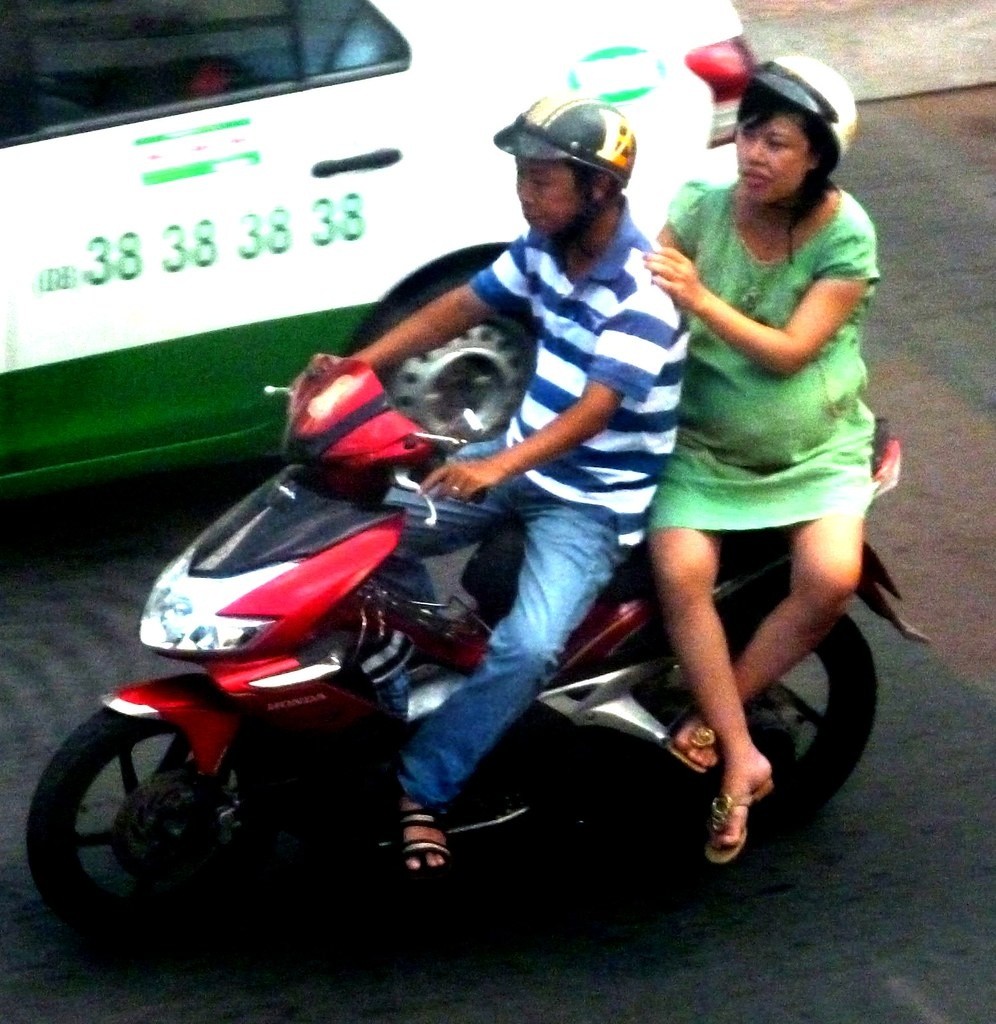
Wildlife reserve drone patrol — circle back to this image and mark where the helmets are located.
[495,93,636,189]
[754,50,858,160]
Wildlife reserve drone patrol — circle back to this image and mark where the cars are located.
[0,1,765,508]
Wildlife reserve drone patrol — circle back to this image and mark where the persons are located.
[314,96,689,881]
[643,54,877,862]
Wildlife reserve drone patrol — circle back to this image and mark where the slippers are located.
[705,776,775,864]
[665,715,722,773]
[385,793,452,880]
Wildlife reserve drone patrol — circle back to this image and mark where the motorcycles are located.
[25,349,933,934]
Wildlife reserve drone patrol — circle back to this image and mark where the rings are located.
[450,485,461,493]
